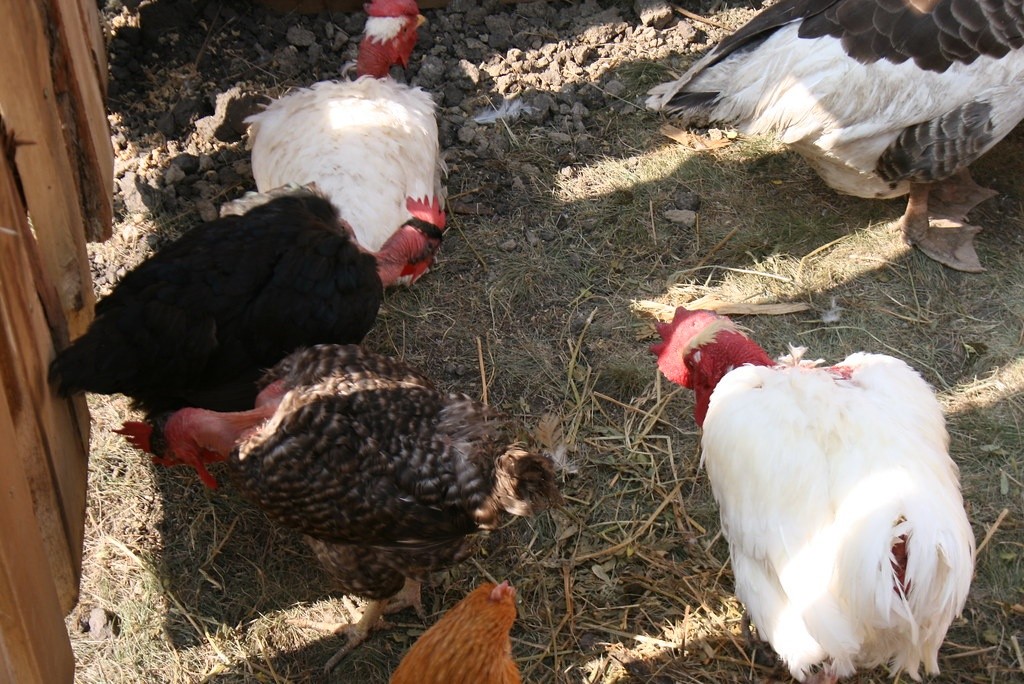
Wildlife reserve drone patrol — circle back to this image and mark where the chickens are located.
[387,579,523,684]
[648,305,978,684]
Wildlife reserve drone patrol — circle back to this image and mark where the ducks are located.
[644,0,1024,274]
